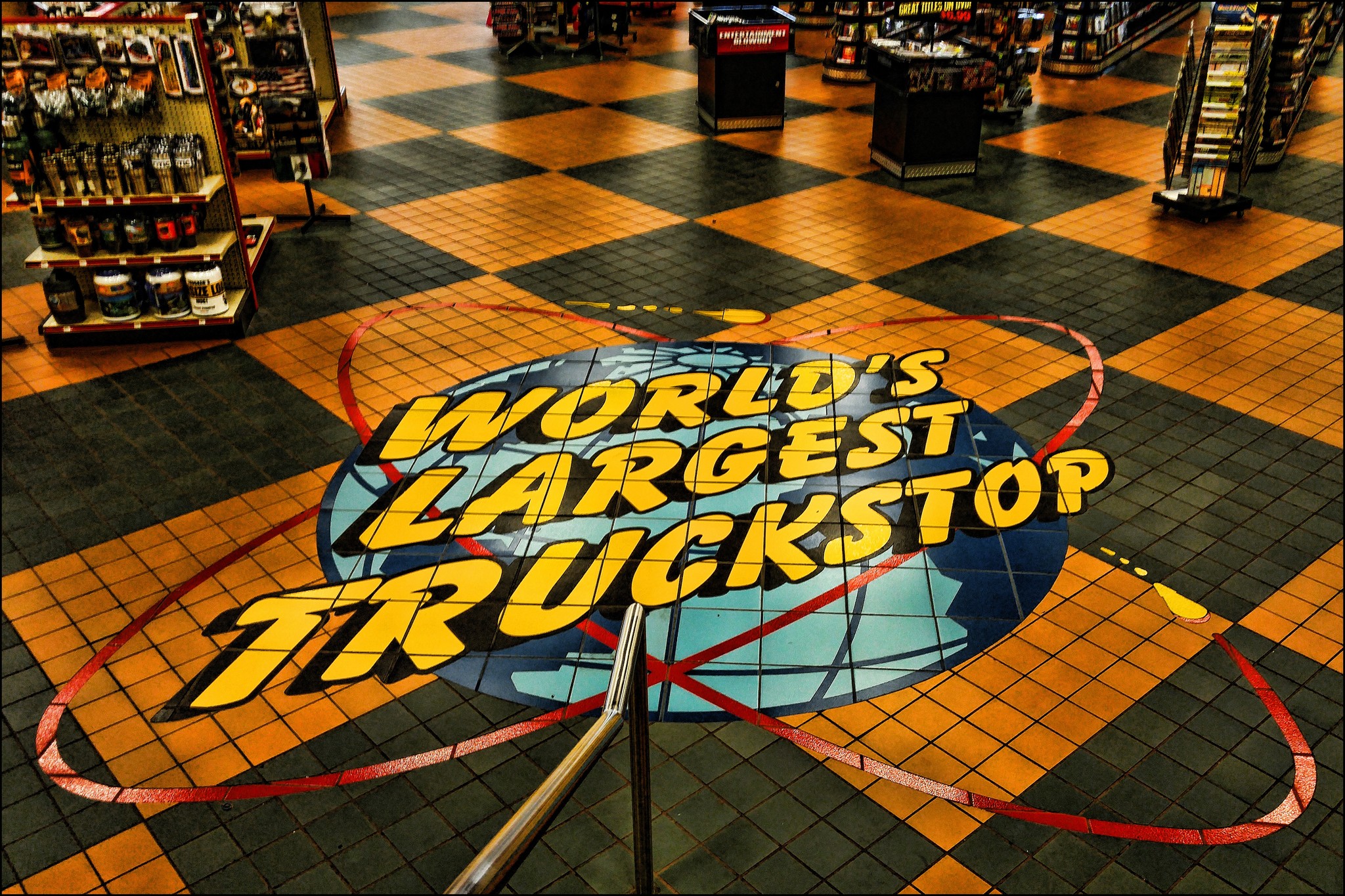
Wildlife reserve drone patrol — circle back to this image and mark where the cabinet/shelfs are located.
[821,2,961,84]
[1254,1,1344,173]
[1041,3,1201,79]
[0,17,276,345]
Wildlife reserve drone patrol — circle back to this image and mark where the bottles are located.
[97,207,125,254]
[153,204,206,253]
[30,207,65,251]
[93,265,142,321]
[145,264,192,318]
[184,260,230,317]
[43,267,87,325]
[58,208,97,257]
[125,205,153,254]
[41,134,206,197]
